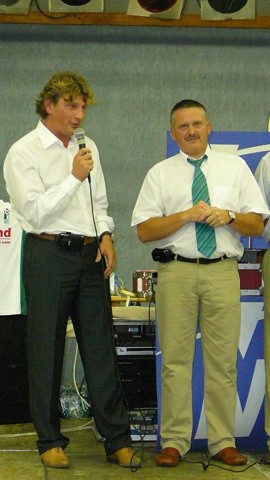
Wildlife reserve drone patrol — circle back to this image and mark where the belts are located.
[33,232,95,245]
[172,253,227,265]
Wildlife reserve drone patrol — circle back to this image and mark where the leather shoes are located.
[155,448,180,466]
[41,446,69,468]
[213,447,248,465]
[107,446,141,467]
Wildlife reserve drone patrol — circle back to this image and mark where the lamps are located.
[197,0,257,20]
[126,0,191,20]
[48,0,105,13]
[0,0,35,15]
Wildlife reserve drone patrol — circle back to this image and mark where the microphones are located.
[74,127,91,182]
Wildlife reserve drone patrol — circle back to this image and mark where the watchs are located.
[99,231,115,244]
[227,210,235,224]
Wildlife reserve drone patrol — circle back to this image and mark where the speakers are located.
[0,314,34,424]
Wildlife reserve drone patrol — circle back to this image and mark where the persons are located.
[1,72,141,468]
[253,153,270,464]
[131,99,270,466]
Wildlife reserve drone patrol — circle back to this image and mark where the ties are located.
[187,154,217,258]
